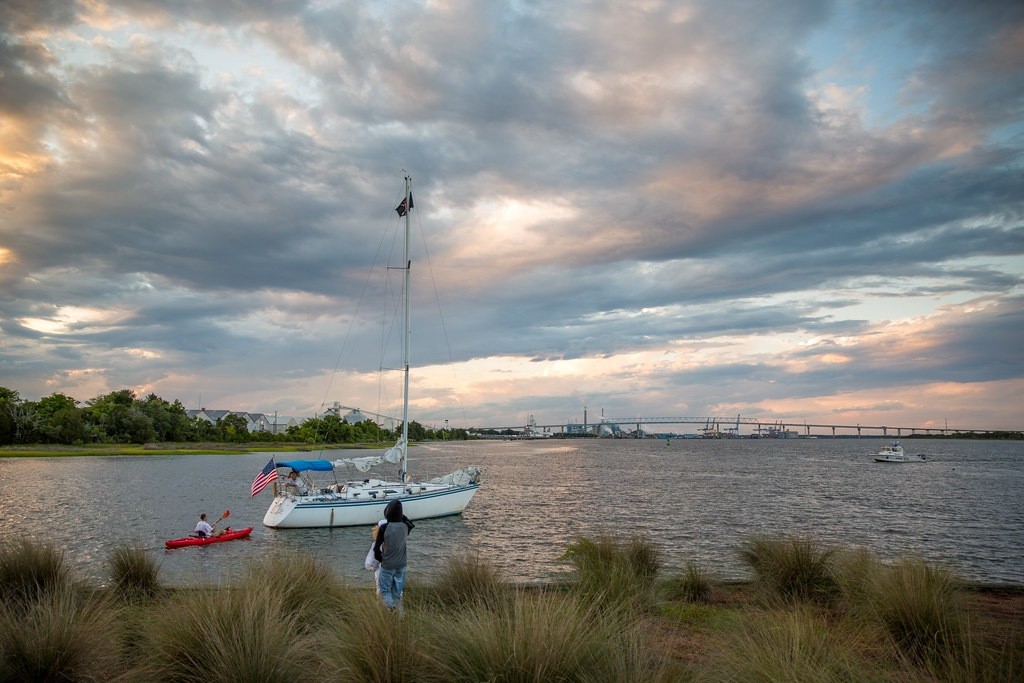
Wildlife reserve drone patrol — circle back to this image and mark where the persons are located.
[194,514,227,538]
[364,499,415,622]
[284,468,308,496]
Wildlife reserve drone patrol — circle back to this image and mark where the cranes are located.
[696,413,742,435]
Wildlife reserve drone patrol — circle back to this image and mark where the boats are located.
[868,441,930,463]
[165,526,255,548]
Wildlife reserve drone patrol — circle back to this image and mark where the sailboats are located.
[262,170,487,529]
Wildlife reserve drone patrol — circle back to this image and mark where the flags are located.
[248,457,279,498]
[395,190,414,217]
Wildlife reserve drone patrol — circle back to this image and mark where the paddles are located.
[211,510,230,526]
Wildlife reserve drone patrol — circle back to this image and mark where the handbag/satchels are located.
[365,541,382,571]
